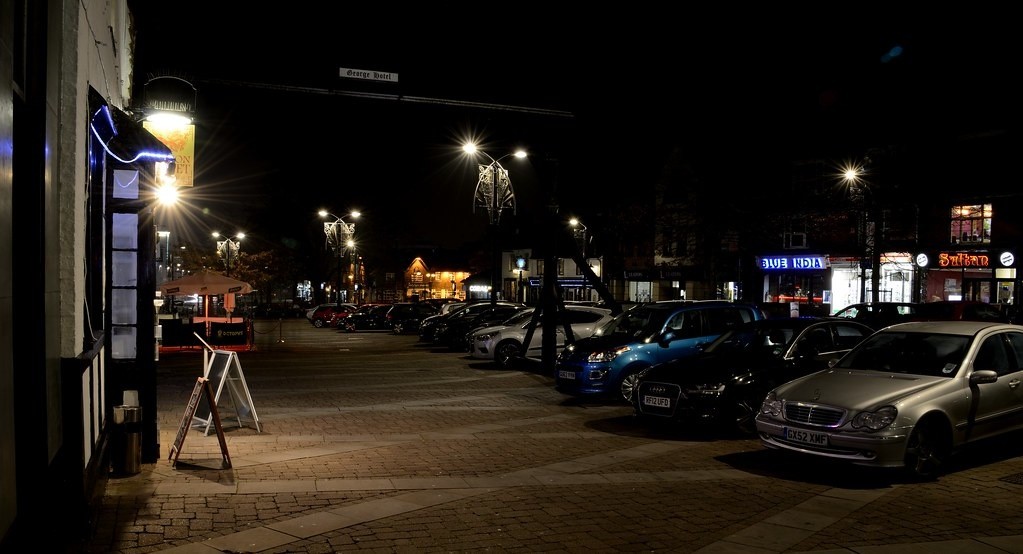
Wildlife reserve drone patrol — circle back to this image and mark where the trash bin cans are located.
[112,406,142,475]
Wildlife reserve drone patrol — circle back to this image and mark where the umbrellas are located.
[160,268,253,338]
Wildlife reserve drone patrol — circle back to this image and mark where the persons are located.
[978,230,989,238]
[267,292,275,311]
[931,290,941,302]
[306,292,308,299]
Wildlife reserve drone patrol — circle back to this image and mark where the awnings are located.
[90,84,175,166]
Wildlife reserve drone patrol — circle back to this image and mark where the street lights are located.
[317,207,363,302]
[570,217,589,266]
[213,231,244,277]
[463,139,532,300]
[842,164,883,304]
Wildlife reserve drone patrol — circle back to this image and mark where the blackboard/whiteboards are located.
[172,377,232,457]
[194,351,258,423]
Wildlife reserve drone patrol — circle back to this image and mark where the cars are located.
[837,300,1011,330]
[553,297,766,403]
[469,305,616,369]
[633,315,883,430]
[306,296,528,346]
[756,322,1023,476]
